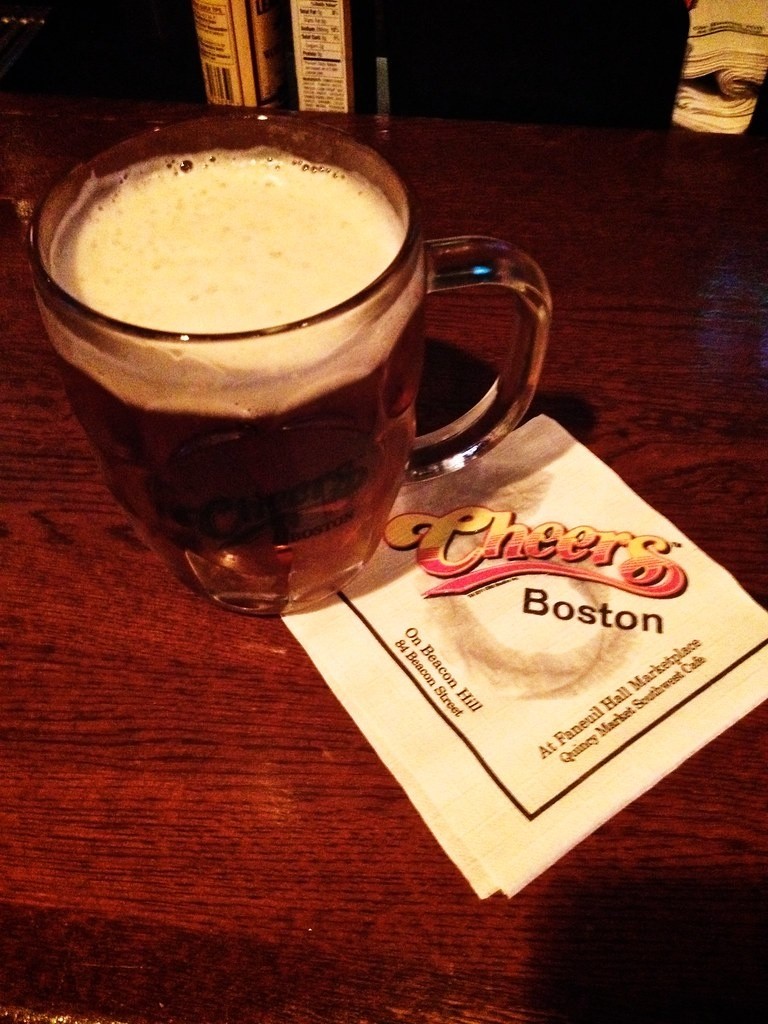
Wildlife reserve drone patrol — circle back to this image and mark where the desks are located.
[0,91,767,1024]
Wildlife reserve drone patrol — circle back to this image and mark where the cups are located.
[26,107,552,615]
